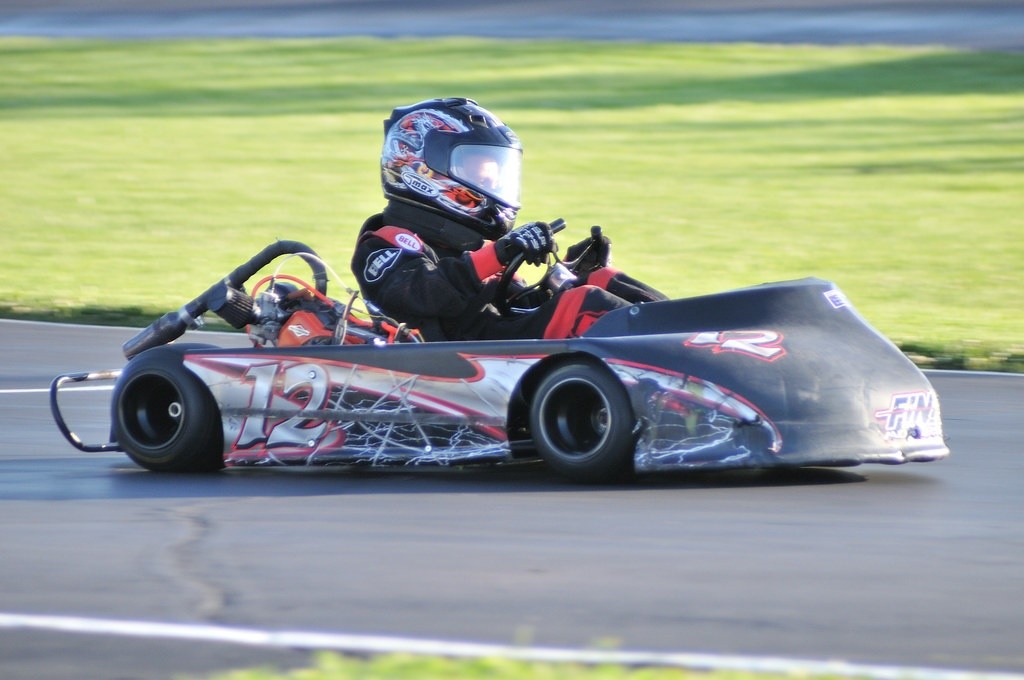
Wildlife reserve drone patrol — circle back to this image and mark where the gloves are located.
[567,234,612,273]
[494,222,559,266]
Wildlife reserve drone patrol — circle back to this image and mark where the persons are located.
[352,96,671,341]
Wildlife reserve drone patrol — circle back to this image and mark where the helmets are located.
[379,96,523,240]
